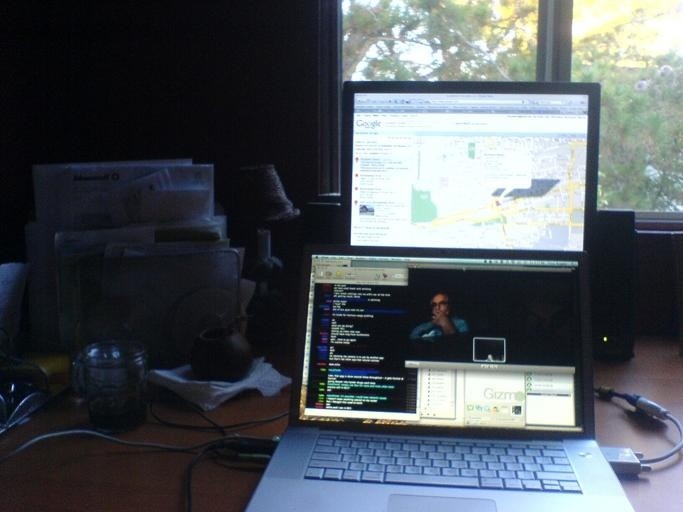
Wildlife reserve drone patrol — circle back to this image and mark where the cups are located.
[72,339,149,434]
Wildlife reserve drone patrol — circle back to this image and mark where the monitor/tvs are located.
[339,80,601,256]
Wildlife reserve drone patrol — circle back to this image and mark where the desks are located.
[0,332,683,512]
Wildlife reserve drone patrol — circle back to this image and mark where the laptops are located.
[242,245,636,512]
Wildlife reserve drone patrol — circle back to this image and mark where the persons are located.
[410,287,475,365]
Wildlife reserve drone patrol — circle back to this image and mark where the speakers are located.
[593,209,636,361]
[303,200,341,246]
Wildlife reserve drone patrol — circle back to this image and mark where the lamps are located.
[233,162,301,283]
